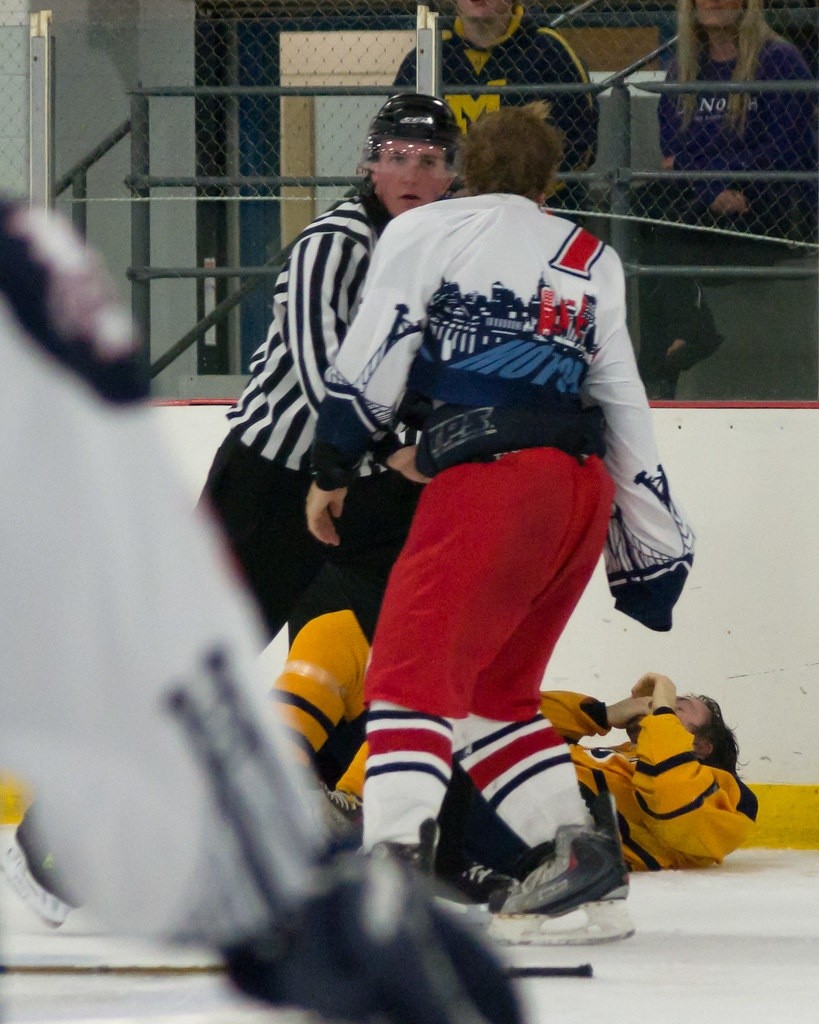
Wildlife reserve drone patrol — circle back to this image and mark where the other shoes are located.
[636,324,726,379]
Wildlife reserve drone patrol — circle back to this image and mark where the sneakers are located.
[434,842,519,915]
[5,799,85,927]
[489,823,634,949]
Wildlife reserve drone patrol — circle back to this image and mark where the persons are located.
[192,92,467,721]
[302,112,690,942]
[267,606,760,914]
[623,1,815,405]
[382,1,598,221]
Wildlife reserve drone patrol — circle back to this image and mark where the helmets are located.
[361,90,462,180]
